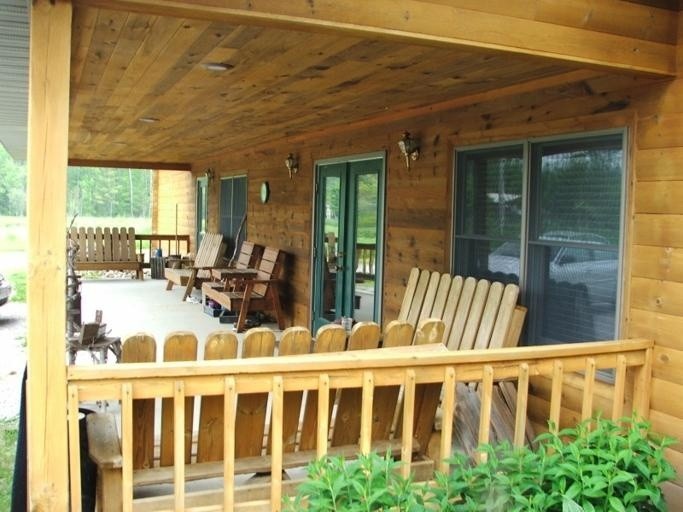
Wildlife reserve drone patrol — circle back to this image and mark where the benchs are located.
[346,266,529,433]
[86,320,450,512]
[66,226,143,280]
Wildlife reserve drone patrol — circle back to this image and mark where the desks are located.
[68,336,122,409]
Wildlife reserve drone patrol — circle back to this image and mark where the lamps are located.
[285,152,298,179]
[397,129,420,169]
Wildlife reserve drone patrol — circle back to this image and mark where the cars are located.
[488,229,618,314]
[0,272,12,305]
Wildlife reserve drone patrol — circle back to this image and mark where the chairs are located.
[201,240,286,334]
[162,232,224,302]
